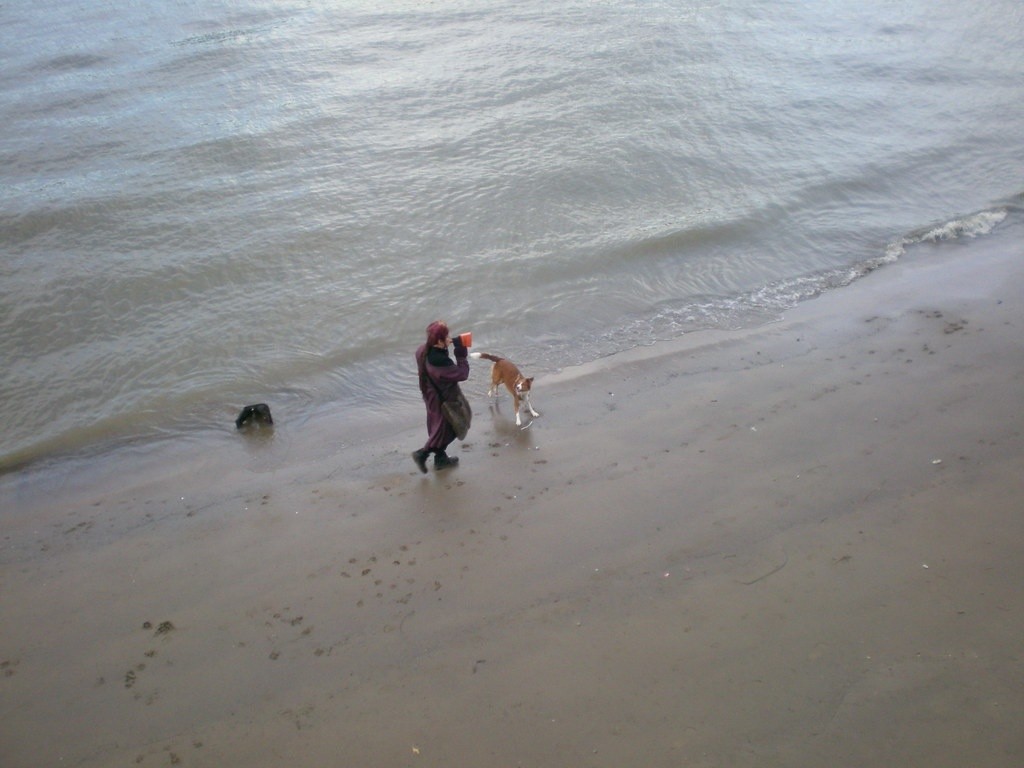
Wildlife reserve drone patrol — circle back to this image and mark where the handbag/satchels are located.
[439,385,473,442]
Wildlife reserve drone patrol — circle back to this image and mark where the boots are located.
[411,450,430,474]
[433,451,459,471]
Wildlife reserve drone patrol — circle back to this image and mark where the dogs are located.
[471,353,539,426]
[236,403,273,425]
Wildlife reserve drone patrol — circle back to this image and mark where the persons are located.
[410,320,469,475]
